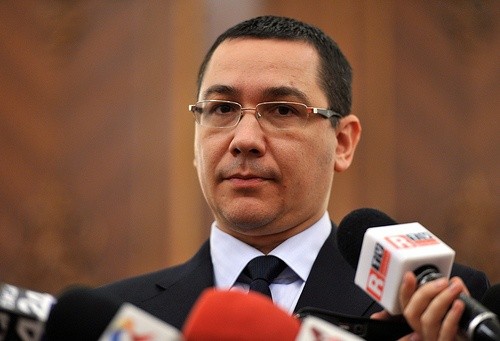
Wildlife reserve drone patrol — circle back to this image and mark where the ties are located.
[242,255,287,303]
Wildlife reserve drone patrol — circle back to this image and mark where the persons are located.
[90,15,491,341]
[398,271,487,341]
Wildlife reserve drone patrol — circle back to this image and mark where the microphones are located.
[0,208,500,341]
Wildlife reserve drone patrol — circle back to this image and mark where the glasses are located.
[189,100,342,129]
